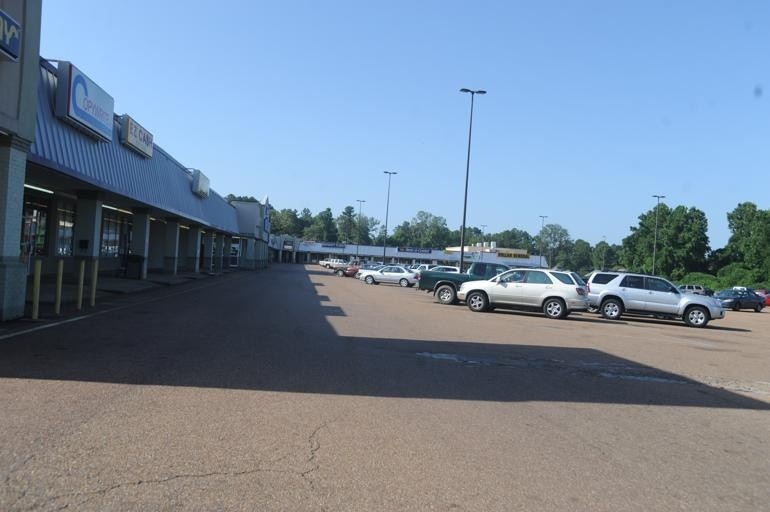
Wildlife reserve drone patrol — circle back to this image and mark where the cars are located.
[457,268,590,319]
[589,271,770,328]
[319,258,460,287]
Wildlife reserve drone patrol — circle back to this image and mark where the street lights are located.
[539,215,547,268]
[357,200,366,256]
[384,171,398,264]
[459,88,487,273]
[652,195,665,275]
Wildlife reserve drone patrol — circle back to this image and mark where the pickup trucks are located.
[418,261,512,304]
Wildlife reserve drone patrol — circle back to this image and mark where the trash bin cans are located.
[126,255,144,279]
